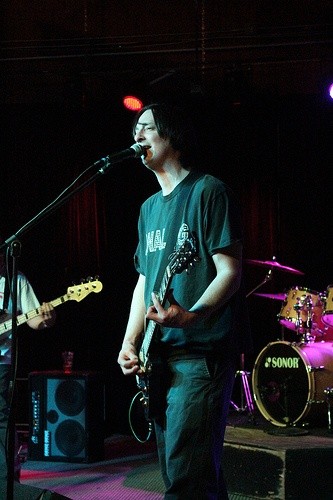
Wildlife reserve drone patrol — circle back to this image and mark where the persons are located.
[0,230,53,482]
[117,104,246,500]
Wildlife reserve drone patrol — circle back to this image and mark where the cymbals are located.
[254,292,287,301]
[246,259,304,275]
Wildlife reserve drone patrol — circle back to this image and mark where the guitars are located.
[131,239,198,409]
[0,279,104,346]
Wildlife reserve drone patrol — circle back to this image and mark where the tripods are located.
[228,355,254,426]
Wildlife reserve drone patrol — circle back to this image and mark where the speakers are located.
[39,374,105,463]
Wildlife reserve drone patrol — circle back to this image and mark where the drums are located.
[252,341,333,428]
[277,286,325,335]
[314,310,333,343]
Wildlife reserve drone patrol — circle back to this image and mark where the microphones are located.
[95,143,144,165]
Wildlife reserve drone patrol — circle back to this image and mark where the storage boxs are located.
[223,426,333,500]
